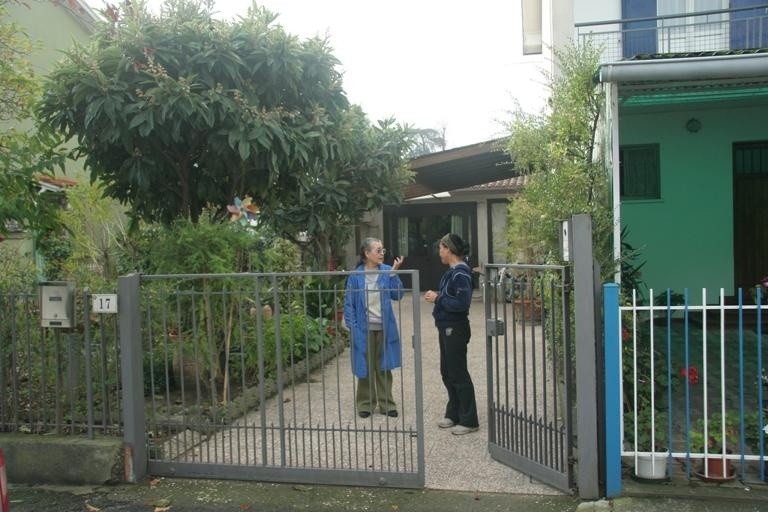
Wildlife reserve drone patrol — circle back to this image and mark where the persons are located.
[425,233,480,435]
[342,236,404,417]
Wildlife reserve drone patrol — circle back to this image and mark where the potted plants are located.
[504,193,547,324]
[623,406,672,484]
[326,217,352,275]
[687,409,762,483]
[146,205,252,388]
[651,289,685,320]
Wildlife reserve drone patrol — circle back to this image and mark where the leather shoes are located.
[382,408,398,418]
[358,408,370,418]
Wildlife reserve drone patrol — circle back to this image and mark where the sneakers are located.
[450,424,479,435]
[436,417,458,428]
